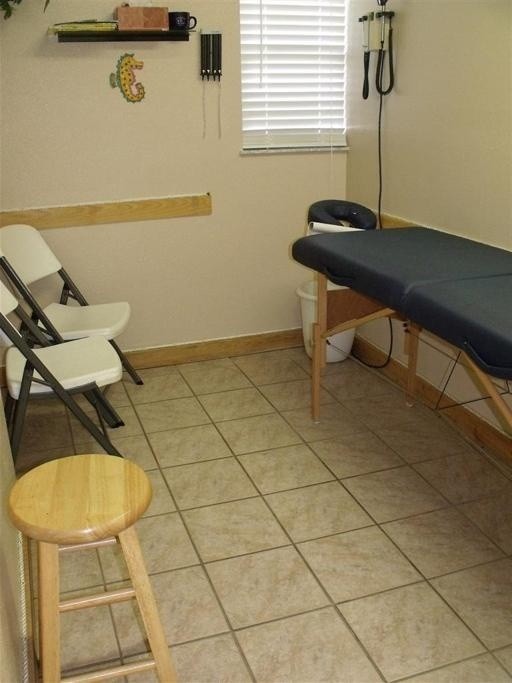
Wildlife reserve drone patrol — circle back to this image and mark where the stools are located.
[9,454,178,683]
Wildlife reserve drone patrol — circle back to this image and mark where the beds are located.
[292,227,512,435]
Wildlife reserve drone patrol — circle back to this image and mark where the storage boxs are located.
[115,7,169,30]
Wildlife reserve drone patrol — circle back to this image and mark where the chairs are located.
[0,223,144,427]
[0,279,123,463]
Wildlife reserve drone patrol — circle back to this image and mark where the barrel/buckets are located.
[295,280,356,363]
